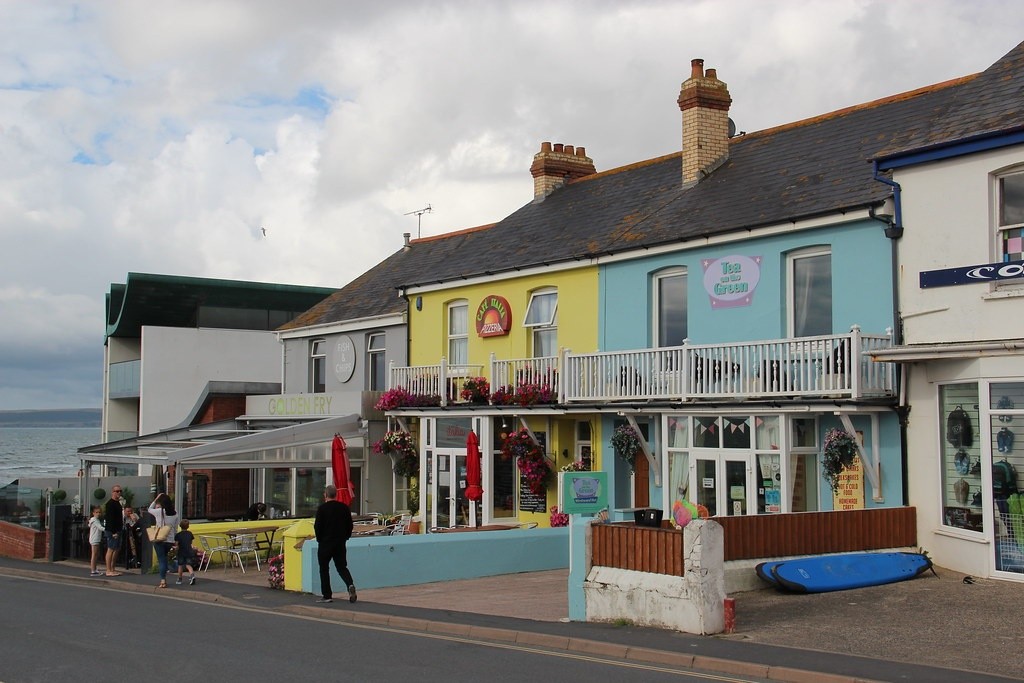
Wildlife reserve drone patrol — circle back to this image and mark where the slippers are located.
[954,451,969,474]
[954,480,969,503]
[997,430,1014,452]
[996,399,1014,421]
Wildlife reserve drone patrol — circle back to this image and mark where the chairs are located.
[431,524,513,532]
[361,513,413,536]
[427,524,471,532]
[273,525,292,554]
[516,521,539,529]
[199,534,261,575]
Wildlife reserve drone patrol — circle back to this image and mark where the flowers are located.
[373,384,442,410]
[560,461,590,473]
[371,430,415,456]
[819,426,859,495]
[608,424,640,475]
[550,505,569,527]
[459,365,559,409]
[502,426,557,497]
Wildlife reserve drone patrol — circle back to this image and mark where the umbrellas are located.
[464,429,485,526]
[331,431,356,505]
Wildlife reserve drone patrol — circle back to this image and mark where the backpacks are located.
[947,406,973,446]
[993,458,1017,498]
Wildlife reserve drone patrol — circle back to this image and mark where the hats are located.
[971,491,982,505]
[970,462,980,473]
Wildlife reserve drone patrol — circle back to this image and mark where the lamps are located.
[502,416,508,428]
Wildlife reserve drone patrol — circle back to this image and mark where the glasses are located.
[113,490,122,493]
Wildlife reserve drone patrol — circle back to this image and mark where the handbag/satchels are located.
[146,508,170,543]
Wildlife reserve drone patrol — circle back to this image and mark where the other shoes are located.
[189,576,195,584]
[91,571,103,577]
[106,570,123,576]
[176,578,182,584]
[160,580,167,587]
[348,585,357,603]
[316,595,333,602]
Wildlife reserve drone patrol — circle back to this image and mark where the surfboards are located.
[771,552,934,593]
[755,559,807,592]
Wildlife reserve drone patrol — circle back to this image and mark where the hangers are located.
[955,405,963,412]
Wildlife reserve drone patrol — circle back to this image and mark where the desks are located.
[696,356,740,394]
[224,526,279,567]
[353,524,387,536]
[352,515,374,523]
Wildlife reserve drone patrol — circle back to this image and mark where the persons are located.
[121,504,141,565]
[104,485,123,576]
[174,519,195,585]
[314,485,358,603]
[247,502,267,520]
[88,507,106,576]
[148,493,179,588]
